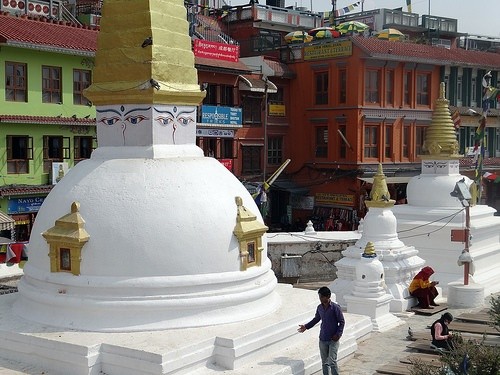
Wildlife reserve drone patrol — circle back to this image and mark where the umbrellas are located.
[285,21,404,41]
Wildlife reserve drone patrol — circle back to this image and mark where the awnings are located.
[0,210,14,230]
[360,176,416,184]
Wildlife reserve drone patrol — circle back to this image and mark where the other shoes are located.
[431,301,439,306]
[421,304,434,309]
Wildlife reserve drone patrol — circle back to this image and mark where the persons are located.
[281,211,305,232]
[298,286,345,375]
[431,312,455,351]
[409,266,440,309]
